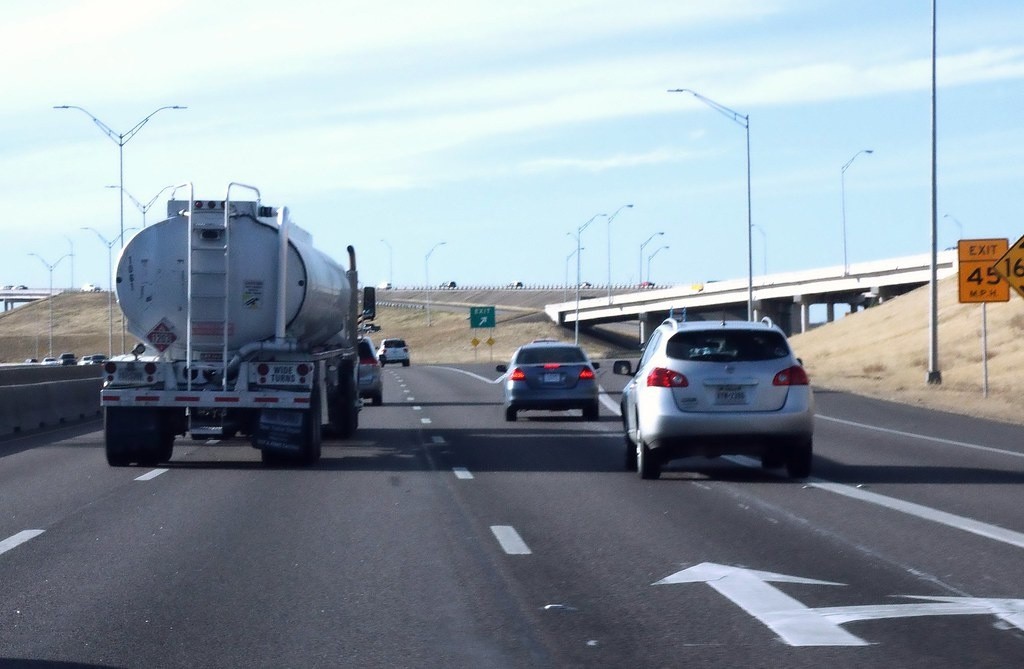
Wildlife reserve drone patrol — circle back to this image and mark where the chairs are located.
[668,342,691,360]
[735,340,766,358]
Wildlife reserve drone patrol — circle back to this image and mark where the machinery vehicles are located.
[99,180,375,463]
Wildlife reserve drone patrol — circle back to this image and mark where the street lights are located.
[80,225,140,357]
[53,101,187,356]
[574,213,606,345]
[607,204,634,305]
[565,249,585,302]
[941,214,964,244]
[647,245,670,288]
[667,87,752,323]
[378,238,394,290]
[104,183,176,228]
[425,240,447,330]
[28,252,74,358]
[841,148,875,272]
[640,231,664,288]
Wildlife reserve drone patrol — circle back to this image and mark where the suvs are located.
[375,339,410,367]
[439,281,456,290]
[353,337,385,405]
[612,317,815,482]
[377,282,391,291]
[496,343,601,421]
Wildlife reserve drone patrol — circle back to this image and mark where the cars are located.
[81,283,101,292]
[638,281,653,288]
[580,282,591,288]
[508,281,522,287]
[12,284,28,290]
[22,353,108,367]
